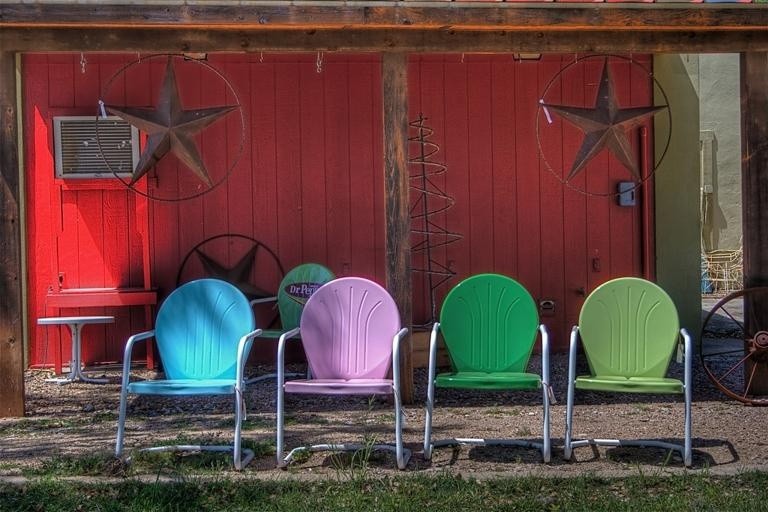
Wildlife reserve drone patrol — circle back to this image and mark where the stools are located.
[38,314,115,384]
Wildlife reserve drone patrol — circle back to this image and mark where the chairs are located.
[242,262,339,386]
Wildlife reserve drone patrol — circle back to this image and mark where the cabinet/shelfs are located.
[47,285,158,376]
